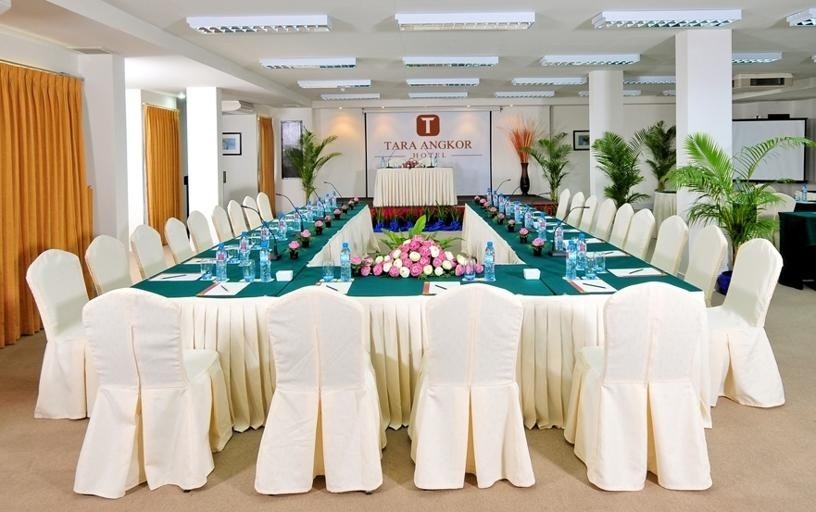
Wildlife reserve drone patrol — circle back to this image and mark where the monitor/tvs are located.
[731,118,808,184]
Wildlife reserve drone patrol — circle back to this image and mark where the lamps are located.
[408,92,468,99]
[320,93,381,101]
[783,8,816,28]
[539,54,641,67]
[406,78,480,88]
[259,57,357,71]
[401,56,499,70]
[511,77,587,86]
[296,79,372,89]
[186,14,334,35]
[495,90,555,99]
[579,90,641,97]
[623,74,676,87]
[394,11,536,32]
[591,8,742,30]
[733,51,782,64]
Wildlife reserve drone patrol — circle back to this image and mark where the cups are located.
[585,257,597,280]
[596,253,606,272]
[201,262,214,281]
[243,260,257,282]
[323,260,335,281]
[464,258,476,280]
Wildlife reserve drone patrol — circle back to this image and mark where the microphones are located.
[324,181,343,206]
[423,147,435,168]
[276,193,304,232]
[524,192,552,214]
[386,146,398,168]
[240,205,282,261]
[505,186,520,204]
[309,187,326,217]
[496,179,511,192]
[549,207,590,256]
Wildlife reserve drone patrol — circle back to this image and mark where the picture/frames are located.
[222,132,242,156]
[573,130,590,151]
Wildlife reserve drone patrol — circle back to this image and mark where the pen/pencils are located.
[327,284,337,292]
[435,283,446,290]
[629,269,644,274]
[602,251,614,255]
[197,258,213,262]
[584,283,606,289]
[220,284,228,292]
[162,273,187,278]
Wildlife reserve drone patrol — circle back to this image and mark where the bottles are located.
[524,206,533,229]
[577,233,588,275]
[801,184,808,200]
[216,242,226,280]
[325,194,330,213]
[331,191,338,209]
[554,221,564,252]
[538,213,547,243]
[307,201,313,222]
[515,201,520,222]
[259,242,271,282]
[506,197,511,215]
[317,197,323,217]
[381,157,385,168]
[566,241,577,280]
[486,188,491,203]
[341,242,352,282]
[434,153,438,167]
[294,208,301,231]
[493,191,498,210]
[278,213,287,240]
[485,241,495,282]
[240,231,250,266]
[260,221,269,247]
[499,193,505,212]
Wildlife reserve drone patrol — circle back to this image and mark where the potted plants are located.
[421,203,435,230]
[495,116,547,195]
[405,206,417,230]
[389,205,402,232]
[448,202,462,230]
[374,201,383,231]
[663,132,816,295]
[434,199,449,229]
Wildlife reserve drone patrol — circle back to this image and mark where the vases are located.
[498,219,503,225]
[343,209,347,213]
[290,251,298,260]
[520,236,527,244]
[325,222,331,228]
[316,229,322,235]
[302,240,309,248]
[533,246,542,256]
[508,225,514,232]
[335,215,340,220]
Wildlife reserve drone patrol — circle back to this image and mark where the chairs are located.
[623,208,655,261]
[683,225,727,307]
[211,206,233,242]
[243,196,262,230]
[578,194,598,233]
[765,192,795,219]
[256,192,274,222]
[564,282,712,492]
[74,288,214,498]
[26,248,90,420]
[608,203,634,250]
[85,234,132,295]
[255,284,382,494]
[131,225,167,278]
[708,238,784,408]
[164,217,193,265]
[187,210,213,253]
[649,215,689,277]
[566,191,585,228]
[407,284,536,489]
[555,188,571,222]
[227,200,248,237]
[590,198,617,242]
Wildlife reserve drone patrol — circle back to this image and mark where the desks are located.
[465,200,704,428]
[777,210,815,289]
[131,202,368,432]
[277,264,555,429]
[794,199,815,211]
[375,166,452,206]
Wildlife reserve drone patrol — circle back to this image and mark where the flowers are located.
[333,209,342,215]
[288,241,300,252]
[348,200,354,204]
[519,228,529,236]
[497,213,505,219]
[489,207,497,212]
[325,215,331,222]
[473,195,480,200]
[351,215,484,279]
[315,220,323,229]
[507,219,515,226]
[354,196,359,201]
[484,202,490,207]
[341,205,348,209]
[480,199,486,204]
[401,158,418,169]
[532,238,545,248]
[300,229,311,240]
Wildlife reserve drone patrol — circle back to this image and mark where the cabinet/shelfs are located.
[501,193,556,216]
